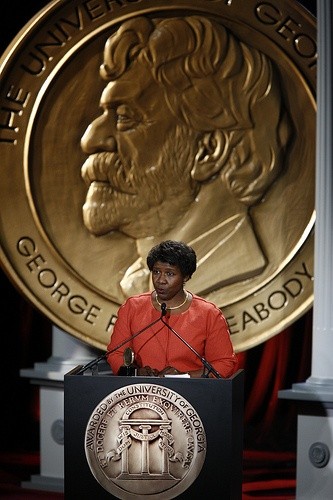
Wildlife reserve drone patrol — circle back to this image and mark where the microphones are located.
[76,303,224,378]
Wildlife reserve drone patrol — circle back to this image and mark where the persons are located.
[107,240,238,379]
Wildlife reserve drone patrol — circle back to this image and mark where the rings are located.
[174,370,178,373]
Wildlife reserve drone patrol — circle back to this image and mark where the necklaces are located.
[156,289,188,310]
